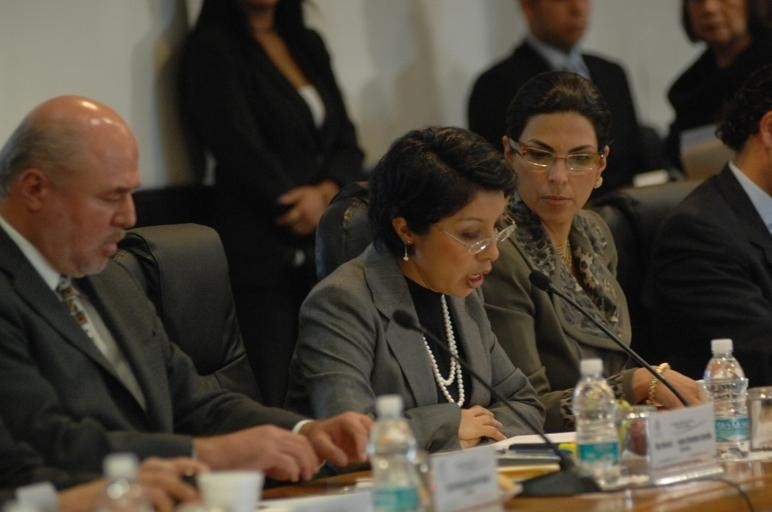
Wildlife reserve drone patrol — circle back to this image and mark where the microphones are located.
[528,266,691,409]
[390,306,596,497]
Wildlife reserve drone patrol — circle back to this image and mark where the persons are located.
[0,456,209,512]
[282,127,546,452]
[0,95,379,491]
[469,2,647,197]
[169,2,366,416]
[483,71,702,432]
[640,70,771,386]
[659,1,772,176]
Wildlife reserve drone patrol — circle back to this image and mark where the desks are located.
[178,391,770,508]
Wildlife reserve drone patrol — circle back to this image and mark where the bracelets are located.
[648,354,668,405]
[112,465,152,512]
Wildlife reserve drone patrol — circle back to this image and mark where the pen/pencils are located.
[508,442,577,450]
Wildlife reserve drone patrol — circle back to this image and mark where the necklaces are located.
[418,291,462,384]
[429,293,467,411]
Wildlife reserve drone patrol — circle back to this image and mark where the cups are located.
[621,404,657,486]
[749,385,772,454]
[194,470,265,512]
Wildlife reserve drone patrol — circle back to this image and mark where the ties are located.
[56,281,112,358]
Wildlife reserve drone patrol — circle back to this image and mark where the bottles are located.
[15,481,61,512]
[703,338,746,484]
[100,452,154,511]
[368,394,420,510]
[573,360,621,488]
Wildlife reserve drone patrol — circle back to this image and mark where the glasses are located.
[509,132,605,171]
[434,212,518,257]
